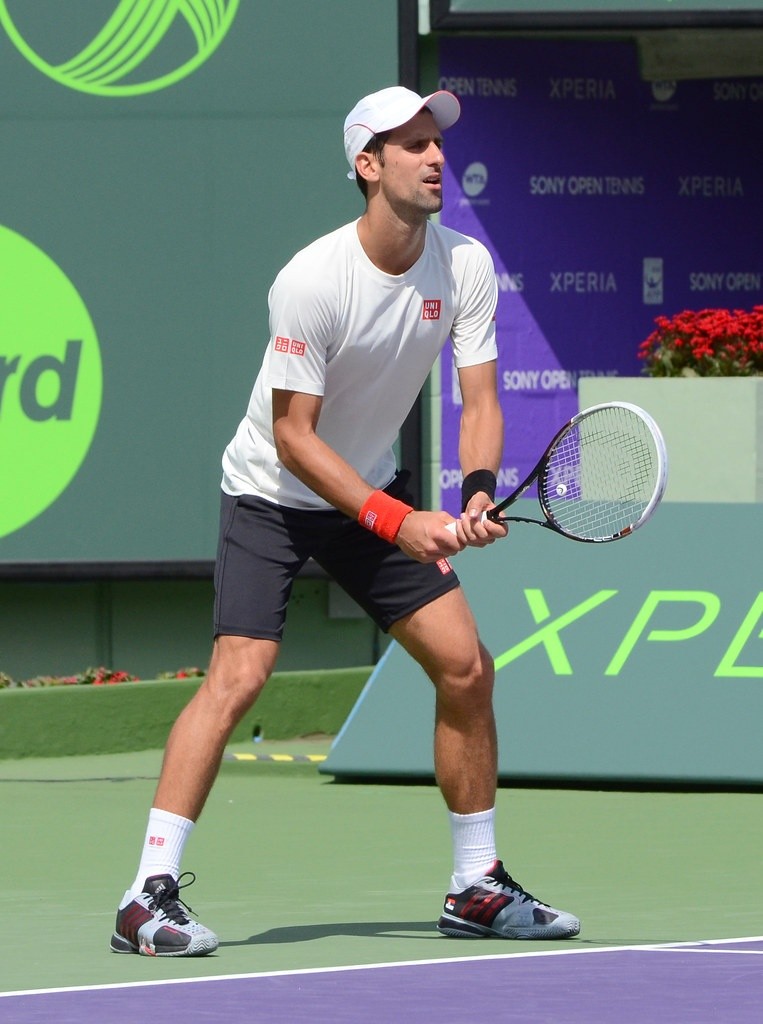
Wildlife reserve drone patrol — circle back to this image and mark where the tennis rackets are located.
[445,402,669,544]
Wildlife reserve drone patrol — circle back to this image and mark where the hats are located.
[342,86,461,182]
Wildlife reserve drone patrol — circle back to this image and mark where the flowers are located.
[639,306,763,378]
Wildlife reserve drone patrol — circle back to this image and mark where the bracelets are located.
[359,490,414,544]
[460,470,497,514]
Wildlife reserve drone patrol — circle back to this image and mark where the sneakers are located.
[437,859,581,941]
[109,875,220,956]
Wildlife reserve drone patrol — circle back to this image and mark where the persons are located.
[110,85,582,958]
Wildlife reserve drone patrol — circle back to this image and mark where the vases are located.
[576,376,763,503]
